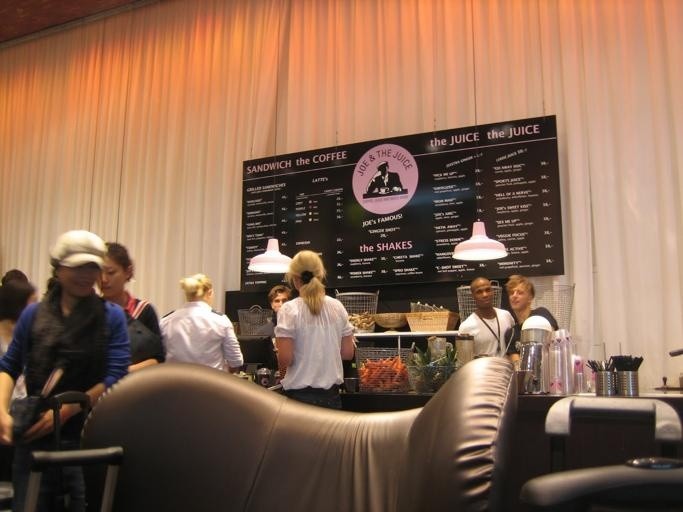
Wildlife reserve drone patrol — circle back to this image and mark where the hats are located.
[50,230,107,269]
[376,161,388,169]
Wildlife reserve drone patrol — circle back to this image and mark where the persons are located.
[505,275,561,367]
[268,286,293,318]
[457,276,516,366]
[0,229,130,511]
[159,274,244,376]
[274,250,355,409]
[364,162,405,196]
[1,279,37,405]
[96,243,165,375]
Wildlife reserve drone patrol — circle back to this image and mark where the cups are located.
[546,330,573,394]
[575,372,586,393]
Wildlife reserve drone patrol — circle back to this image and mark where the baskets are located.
[405,311,460,333]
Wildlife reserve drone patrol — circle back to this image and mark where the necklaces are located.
[476,309,502,351]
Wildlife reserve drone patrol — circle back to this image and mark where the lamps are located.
[452,222,508,261]
[248,239,293,274]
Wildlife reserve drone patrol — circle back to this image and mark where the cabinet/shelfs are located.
[227,334,488,413]
